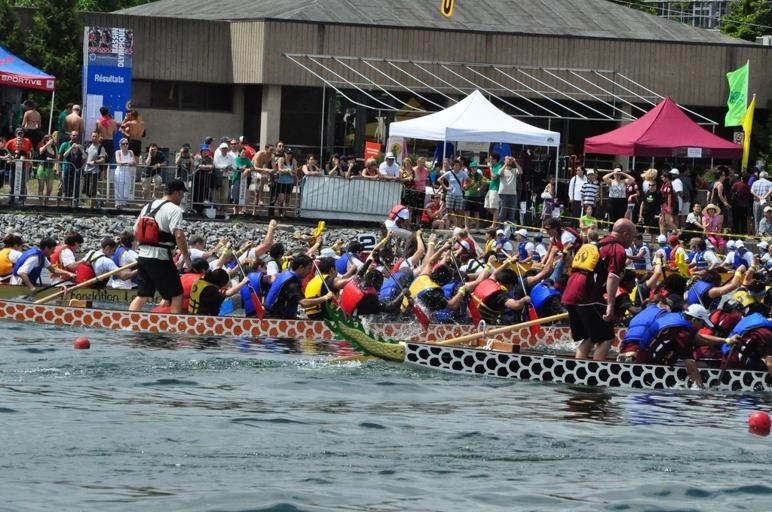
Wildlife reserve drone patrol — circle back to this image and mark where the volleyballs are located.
[74,336,90,349]
[750,412,771,430]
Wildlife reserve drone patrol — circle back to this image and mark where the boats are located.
[0,297,633,359]
[310,290,772,400]
[0,277,165,306]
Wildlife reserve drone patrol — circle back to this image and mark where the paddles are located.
[380,258,430,332]
[514,261,544,335]
[449,251,480,328]
[230,246,266,321]
[15,280,71,301]
[322,306,569,367]
[313,261,341,309]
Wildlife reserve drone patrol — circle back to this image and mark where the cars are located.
[561,165,615,181]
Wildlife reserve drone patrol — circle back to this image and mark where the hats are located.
[685,304,717,328]
[448,219,769,271]
[167,180,189,192]
[201,136,440,179]
[431,194,440,199]
[469,163,477,168]
[569,161,771,221]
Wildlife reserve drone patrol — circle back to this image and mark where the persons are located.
[747,410,771,436]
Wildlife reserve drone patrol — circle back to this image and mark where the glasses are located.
[120,143,129,146]
